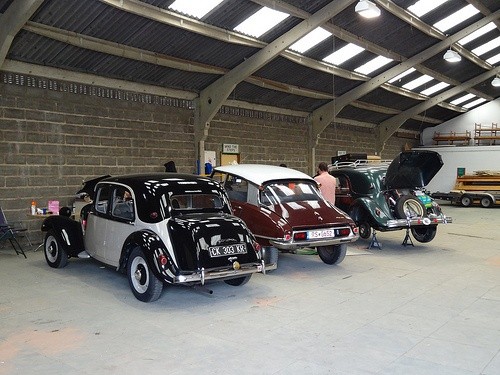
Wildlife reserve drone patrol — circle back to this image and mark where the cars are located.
[312,149,453,243]
[40,172,266,303]
[207,163,361,265]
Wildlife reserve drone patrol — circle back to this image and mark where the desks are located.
[28,212,72,252]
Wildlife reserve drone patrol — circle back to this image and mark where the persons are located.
[313,162,339,206]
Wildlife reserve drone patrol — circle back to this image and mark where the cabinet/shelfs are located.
[473,123,500,146]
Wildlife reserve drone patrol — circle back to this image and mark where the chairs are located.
[0,206,29,258]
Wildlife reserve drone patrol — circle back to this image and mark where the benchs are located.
[431,130,471,146]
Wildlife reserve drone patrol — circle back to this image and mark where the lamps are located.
[492,74,499,87]
[444,49,462,63]
[354,0,382,18]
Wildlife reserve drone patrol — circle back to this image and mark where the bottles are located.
[31,202,35,215]
